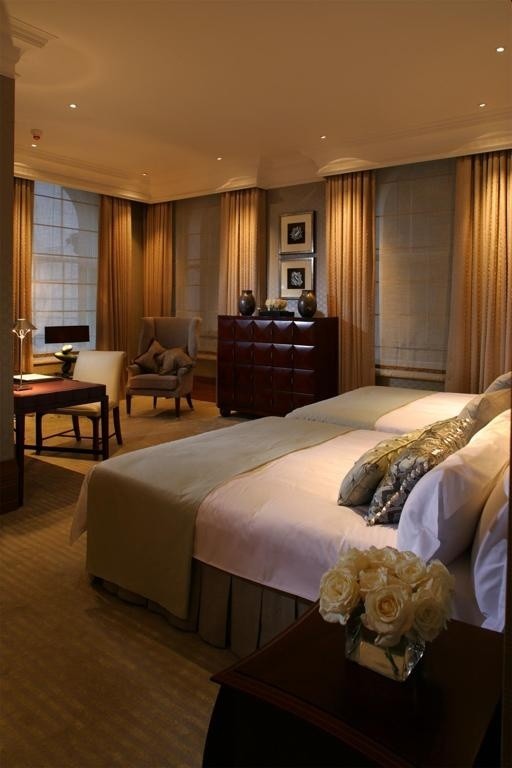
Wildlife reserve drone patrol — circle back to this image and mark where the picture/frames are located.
[277,254,317,301]
[278,209,317,256]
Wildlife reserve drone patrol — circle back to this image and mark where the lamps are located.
[10,316,38,391]
[43,324,90,380]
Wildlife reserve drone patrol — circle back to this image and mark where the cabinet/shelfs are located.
[215,313,340,420]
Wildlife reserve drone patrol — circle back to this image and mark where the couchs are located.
[125,312,202,418]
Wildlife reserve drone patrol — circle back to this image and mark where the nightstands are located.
[199,594,512,767]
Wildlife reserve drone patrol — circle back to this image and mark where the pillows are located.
[336,432,422,509]
[471,464,510,634]
[395,409,511,577]
[149,347,195,374]
[460,389,511,424]
[132,336,164,371]
[368,412,479,524]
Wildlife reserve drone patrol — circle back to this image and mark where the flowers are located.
[318,542,460,643]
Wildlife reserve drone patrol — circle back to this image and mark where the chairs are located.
[35,348,127,456]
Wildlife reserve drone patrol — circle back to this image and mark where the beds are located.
[284,383,512,435]
[64,412,512,660]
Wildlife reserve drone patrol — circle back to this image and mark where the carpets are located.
[0,393,252,765]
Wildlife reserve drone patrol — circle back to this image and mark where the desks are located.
[10,373,112,507]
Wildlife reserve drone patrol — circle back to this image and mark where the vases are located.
[338,619,429,683]
[237,289,256,316]
[297,289,318,318]
